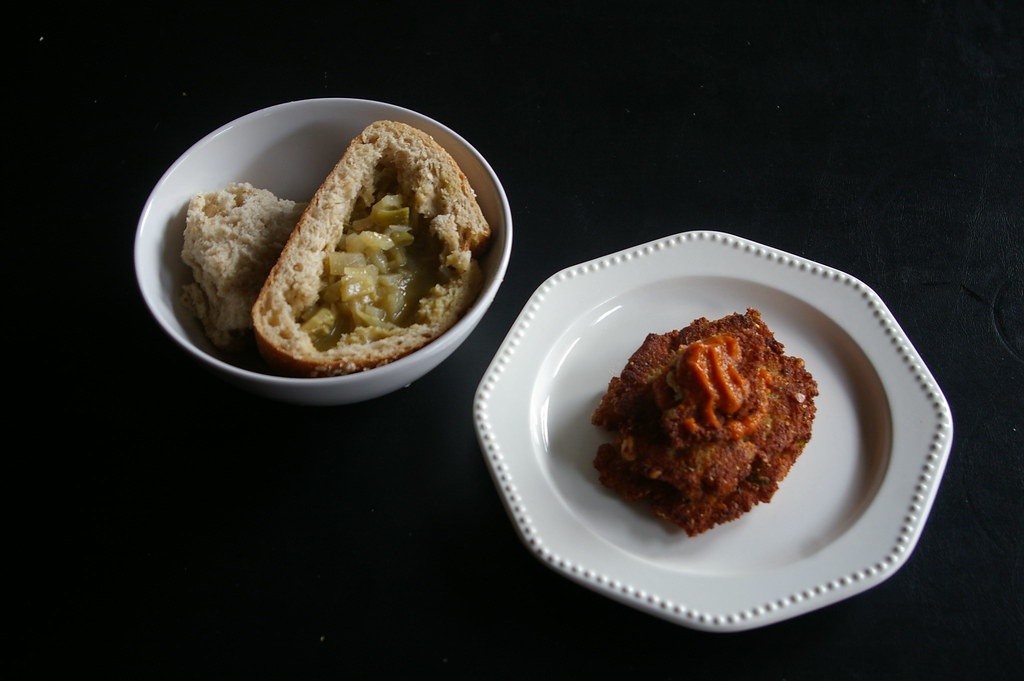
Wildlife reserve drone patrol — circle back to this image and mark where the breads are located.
[182,180,313,350]
[251,120,491,379]
[589,306,819,533]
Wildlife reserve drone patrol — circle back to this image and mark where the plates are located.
[473,232,953,632]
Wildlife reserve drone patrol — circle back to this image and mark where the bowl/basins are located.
[133,96,513,405]
